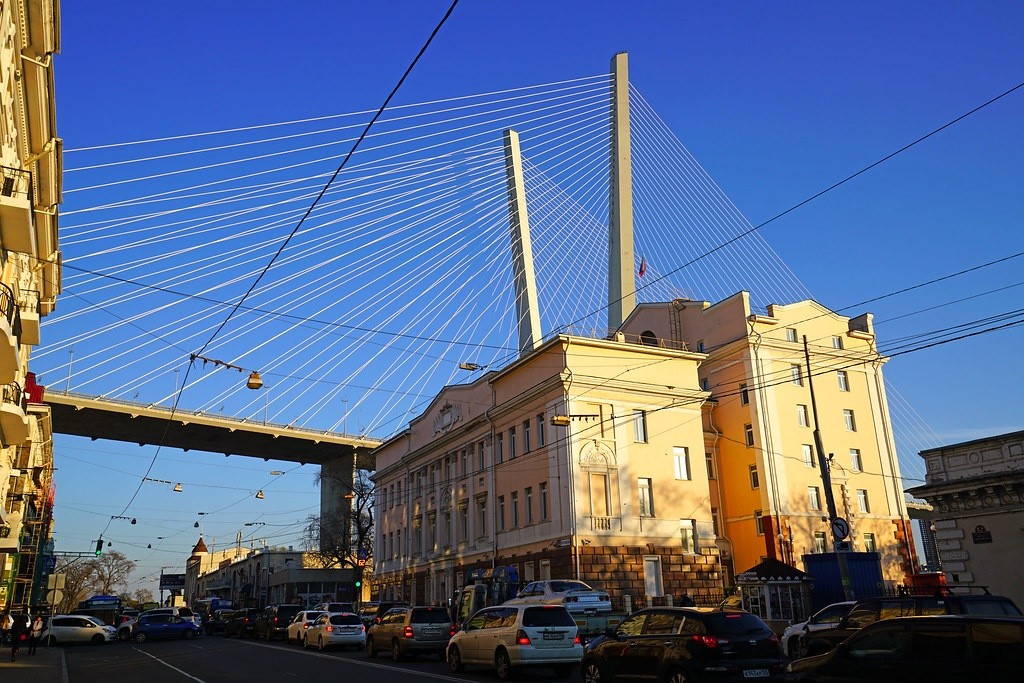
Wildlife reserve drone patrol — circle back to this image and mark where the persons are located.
[28,616,45,656]
[0,611,14,649]
[9,615,26,662]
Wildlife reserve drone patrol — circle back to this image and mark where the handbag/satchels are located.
[20,634,26,640]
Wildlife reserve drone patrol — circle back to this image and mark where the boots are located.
[11,648,19,662]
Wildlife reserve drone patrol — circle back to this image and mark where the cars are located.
[38,614,116,645]
[115,603,457,659]
[446,576,1024,683]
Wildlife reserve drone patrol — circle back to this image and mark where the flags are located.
[639,256,645,276]
